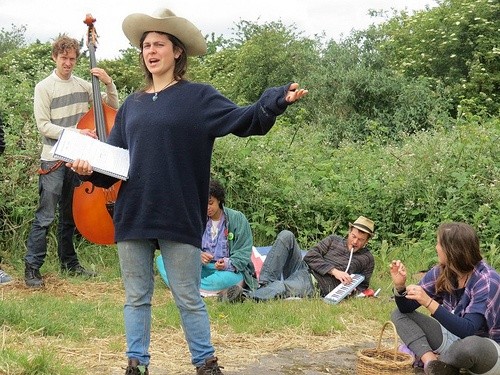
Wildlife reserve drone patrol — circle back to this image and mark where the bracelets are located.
[426,299,432,308]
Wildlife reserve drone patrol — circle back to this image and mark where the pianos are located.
[323,274,365,304]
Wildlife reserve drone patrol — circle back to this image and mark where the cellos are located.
[72,13,123,245]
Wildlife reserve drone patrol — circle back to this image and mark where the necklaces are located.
[152,80,176,101]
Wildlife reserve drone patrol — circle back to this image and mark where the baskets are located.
[356,321,415,375]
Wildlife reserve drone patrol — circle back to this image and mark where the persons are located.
[0,129,15,285]
[155,180,253,296]
[65,8,309,375]
[25,36,120,288]
[390,223,500,375]
[217,215,375,303]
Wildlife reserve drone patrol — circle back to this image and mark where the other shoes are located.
[196,357,225,375]
[121,358,149,375]
[398,344,424,370]
[217,284,246,303]
[425,360,467,374]
[61,264,97,278]
[25,264,45,288]
[0,270,15,286]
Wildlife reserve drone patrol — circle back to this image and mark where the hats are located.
[349,215,380,239]
[122,8,207,57]
